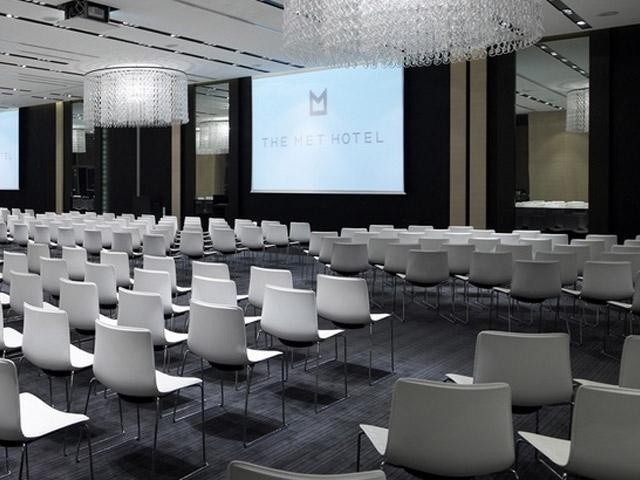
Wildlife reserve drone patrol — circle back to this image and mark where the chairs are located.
[309,224,639,360]
[357,378,520,480]
[248,285,348,413]
[315,274,394,386]
[226,460,387,480]
[515,383,639,480]
[569,334,639,440]
[1,358,94,479]
[442,331,574,463]
[172,298,286,447]
[76,319,206,480]
[1,241,261,375]
[18,301,94,412]
[249,264,293,346]
[1,206,311,277]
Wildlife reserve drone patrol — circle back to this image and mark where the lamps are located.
[78,64,192,136]
[563,88,590,137]
[197,119,230,158]
[280,0,547,74]
[72,129,87,154]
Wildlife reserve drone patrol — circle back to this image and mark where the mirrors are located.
[72,100,98,210]
[194,80,230,214]
[512,32,591,212]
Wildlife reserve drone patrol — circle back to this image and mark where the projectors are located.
[64,1,110,23]
